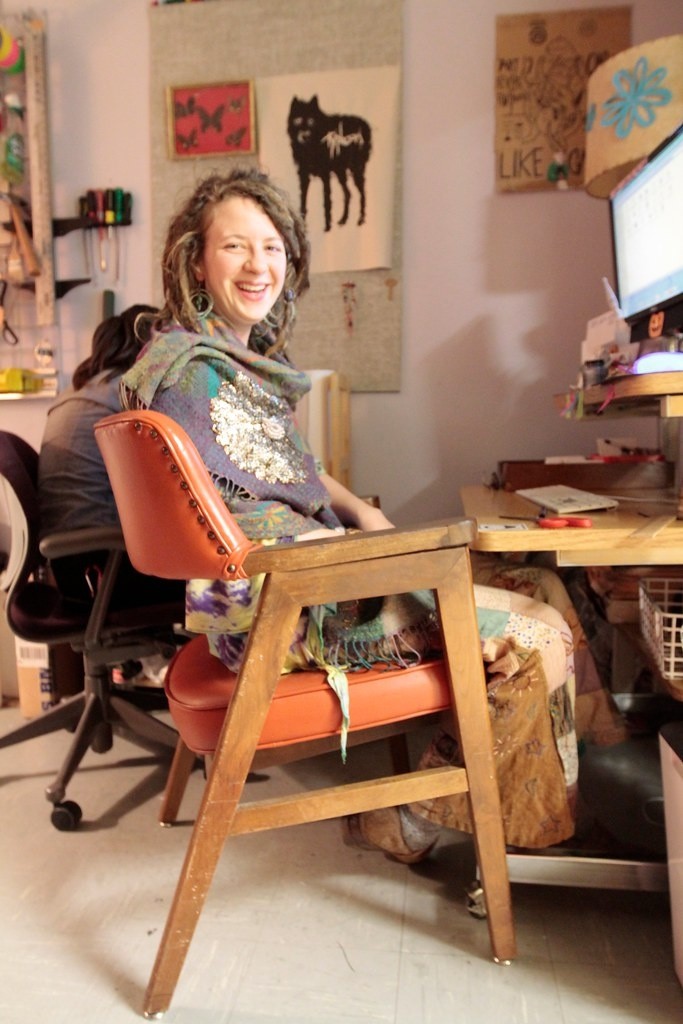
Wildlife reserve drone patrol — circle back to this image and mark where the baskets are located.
[640,576,683,680]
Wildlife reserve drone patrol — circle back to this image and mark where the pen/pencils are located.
[540,506,547,517]
[604,440,635,455]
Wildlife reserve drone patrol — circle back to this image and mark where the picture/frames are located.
[165,76,259,162]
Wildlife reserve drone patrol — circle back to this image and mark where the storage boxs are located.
[14,635,62,721]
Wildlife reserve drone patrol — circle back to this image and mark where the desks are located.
[453,482,683,982]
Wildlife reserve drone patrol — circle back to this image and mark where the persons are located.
[37,306,189,621]
[115,171,628,878]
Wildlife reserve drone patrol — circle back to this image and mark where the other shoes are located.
[341,807,440,864]
[528,812,625,857]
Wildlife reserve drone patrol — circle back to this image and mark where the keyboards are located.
[516,485,619,515]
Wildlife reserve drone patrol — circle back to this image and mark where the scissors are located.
[502,515,591,529]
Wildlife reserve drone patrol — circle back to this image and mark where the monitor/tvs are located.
[608,123,683,343]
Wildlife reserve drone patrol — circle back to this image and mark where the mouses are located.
[631,352,683,375]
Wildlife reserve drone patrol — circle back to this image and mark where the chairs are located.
[90,410,521,1020]
[0,428,203,831]
